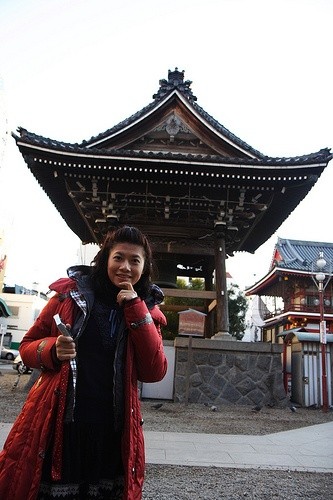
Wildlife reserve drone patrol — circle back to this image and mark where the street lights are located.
[308,250,332,413]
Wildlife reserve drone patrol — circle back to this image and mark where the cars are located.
[0,346,20,360]
[12,354,34,374]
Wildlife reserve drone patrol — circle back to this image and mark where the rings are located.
[124,295,126,300]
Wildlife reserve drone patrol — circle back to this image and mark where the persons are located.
[0,226,168,500]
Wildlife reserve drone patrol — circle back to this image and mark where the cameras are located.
[52,315,73,340]
